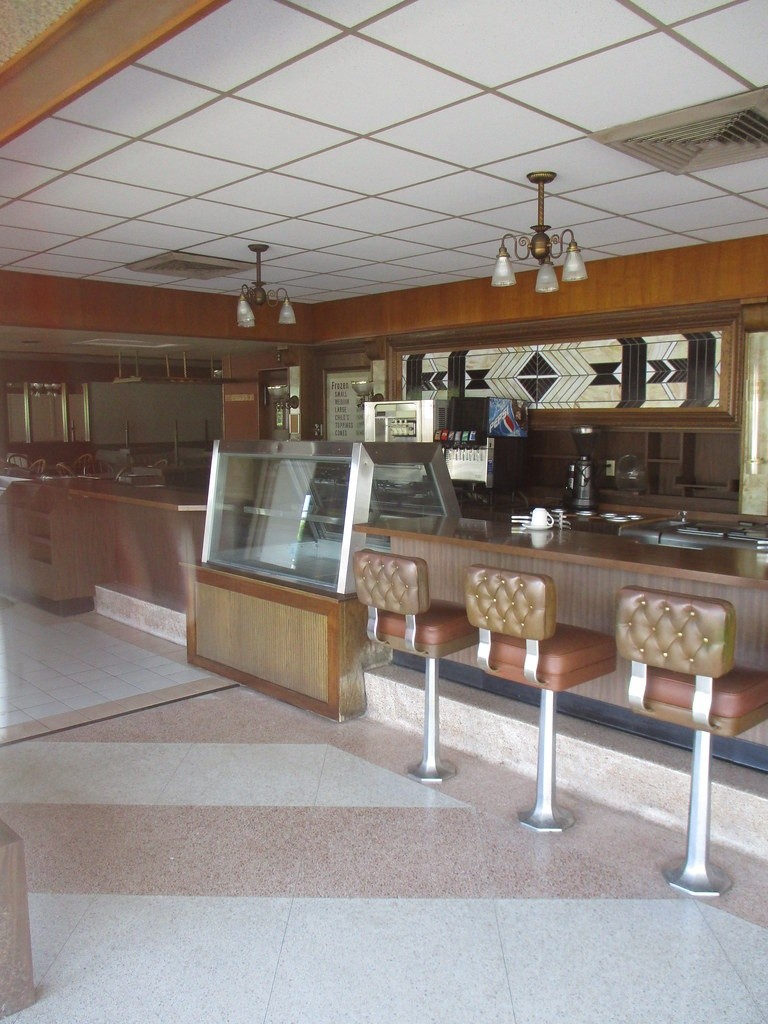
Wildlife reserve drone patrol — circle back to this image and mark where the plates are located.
[524,524,553,530]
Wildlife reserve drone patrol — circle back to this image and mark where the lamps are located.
[351,378,385,411]
[237,244,296,328]
[267,384,299,412]
[491,171,590,293]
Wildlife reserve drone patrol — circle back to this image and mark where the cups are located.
[489,400,521,437]
[529,508,554,527]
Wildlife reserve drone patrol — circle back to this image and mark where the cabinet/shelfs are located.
[200,439,463,595]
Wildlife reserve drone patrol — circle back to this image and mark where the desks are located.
[352,515,768,773]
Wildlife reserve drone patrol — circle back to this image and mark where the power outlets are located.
[605,460,615,476]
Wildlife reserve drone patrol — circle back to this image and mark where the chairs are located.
[613,585,768,898]
[352,548,481,784]
[464,564,617,834]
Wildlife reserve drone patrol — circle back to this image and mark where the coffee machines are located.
[564,424,604,510]
